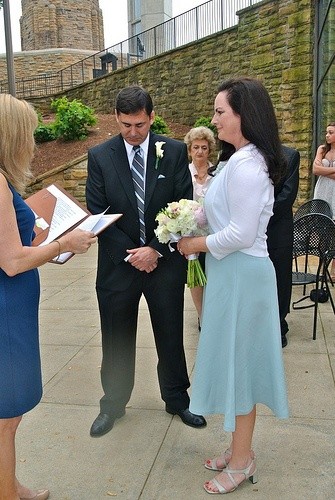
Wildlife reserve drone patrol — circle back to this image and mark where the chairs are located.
[292,198,335,340]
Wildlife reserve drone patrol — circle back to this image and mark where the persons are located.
[312,123,335,283]
[265,144,300,348]
[85,86,208,437]
[176,78,287,495]
[0,94,97,500]
[184,126,216,331]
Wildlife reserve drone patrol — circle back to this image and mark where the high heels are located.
[205,448,256,471]
[202,457,258,494]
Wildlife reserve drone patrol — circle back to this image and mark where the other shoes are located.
[21,488,50,500]
[319,272,334,282]
[281,334,289,348]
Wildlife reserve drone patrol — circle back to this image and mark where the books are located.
[23,184,122,264]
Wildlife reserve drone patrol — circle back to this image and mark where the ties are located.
[129,145,148,247]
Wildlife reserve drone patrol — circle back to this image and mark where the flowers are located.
[193,209,208,232]
[155,140,166,170]
[154,198,207,288]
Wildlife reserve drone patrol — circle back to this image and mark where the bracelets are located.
[54,241,60,261]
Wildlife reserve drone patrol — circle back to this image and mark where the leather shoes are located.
[165,406,207,428]
[90,409,127,438]
